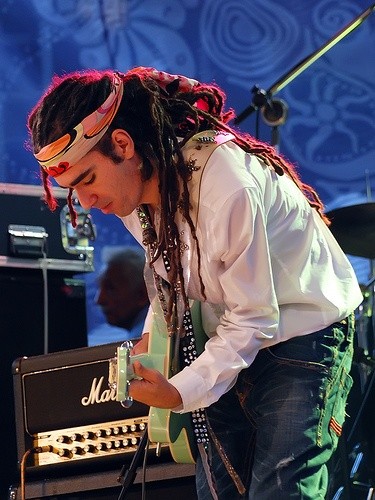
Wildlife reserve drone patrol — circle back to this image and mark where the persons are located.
[95,246,154,340]
[26,65,366,500]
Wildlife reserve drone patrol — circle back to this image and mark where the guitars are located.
[108,292,208,464]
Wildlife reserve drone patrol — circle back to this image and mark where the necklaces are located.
[134,137,189,340]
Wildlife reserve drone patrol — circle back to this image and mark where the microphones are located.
[263,98,288,125]
